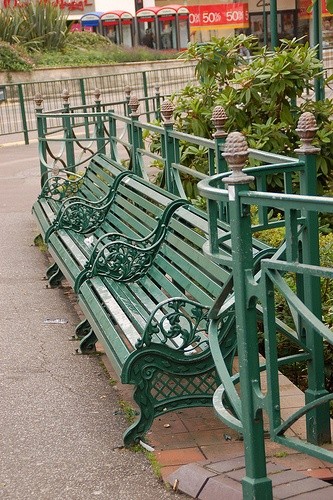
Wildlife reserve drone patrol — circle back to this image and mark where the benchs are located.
[31,153,285,452]
